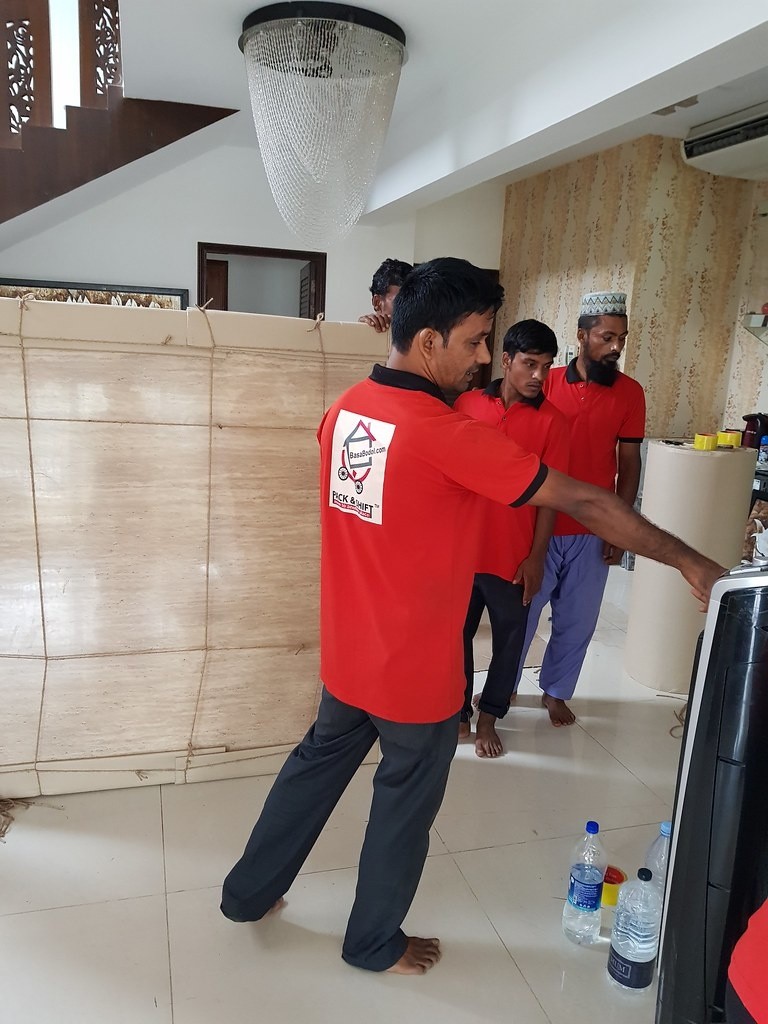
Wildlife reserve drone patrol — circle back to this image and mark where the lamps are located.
[237,1,409,248]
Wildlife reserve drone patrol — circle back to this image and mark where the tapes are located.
[694,432,718,451]
[716,430,742,446]
[601,864,627,905]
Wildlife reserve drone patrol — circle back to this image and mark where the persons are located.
[221,258,727,974]
[725,898,768,1024]
[358,257,645,760]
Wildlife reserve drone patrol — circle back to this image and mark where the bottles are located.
[561,821,607,946]
[642,821,671,902]
[604,868,662,992]
[758,436,768,464]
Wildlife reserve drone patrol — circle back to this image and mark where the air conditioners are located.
[680,101,768,181]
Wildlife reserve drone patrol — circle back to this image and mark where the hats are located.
[580,292,627,316]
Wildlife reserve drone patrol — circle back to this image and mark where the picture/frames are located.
[0,278,189,311]
